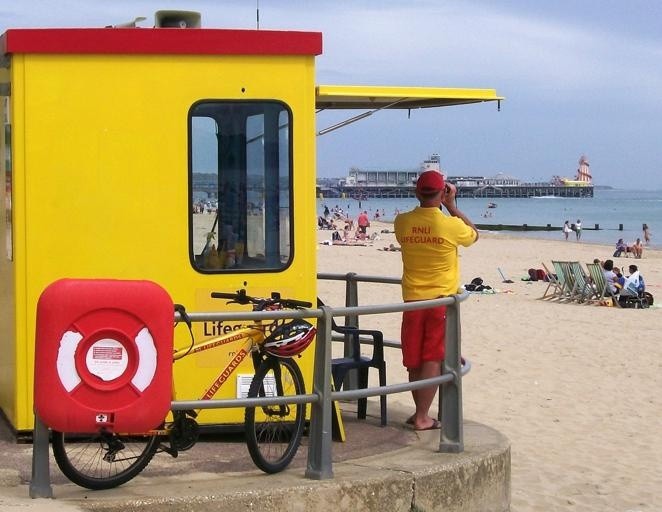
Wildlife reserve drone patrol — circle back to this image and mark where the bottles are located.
[201,222,244,270]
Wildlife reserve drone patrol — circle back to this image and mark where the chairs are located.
[317,298,386,428]
[541,260,649,308]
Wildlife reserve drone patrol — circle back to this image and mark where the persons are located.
[192,200,263,217]
[395,171,478,430]
[317,200,388,244]
[561,219,651,301]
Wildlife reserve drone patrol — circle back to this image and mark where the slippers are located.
[414,416,444,432]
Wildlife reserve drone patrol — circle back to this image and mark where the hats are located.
[417,170,446,194]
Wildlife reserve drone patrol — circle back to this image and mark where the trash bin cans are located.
[595,224,599,229]
[620,224,623,230]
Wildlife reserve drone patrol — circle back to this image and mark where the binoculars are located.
[446,186,458,194]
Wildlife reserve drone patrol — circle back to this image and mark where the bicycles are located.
[47,287,321,488]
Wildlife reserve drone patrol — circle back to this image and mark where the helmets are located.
[259,319,321,360]
